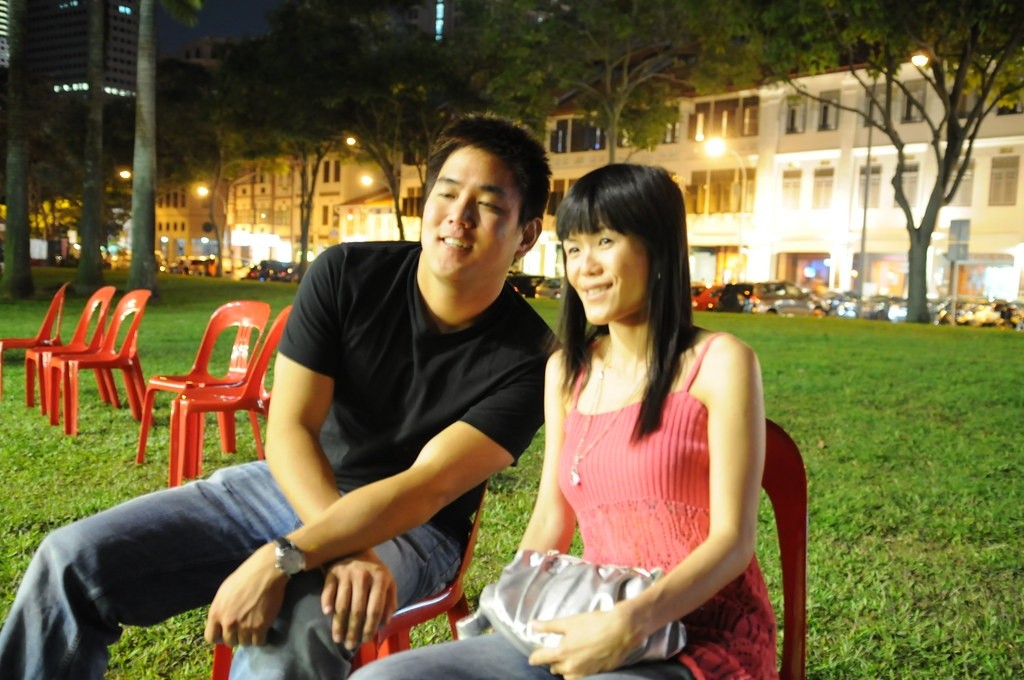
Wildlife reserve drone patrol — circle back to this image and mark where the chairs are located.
[211,469,490,680]
[0,280,292,490]
[761,413,810,680]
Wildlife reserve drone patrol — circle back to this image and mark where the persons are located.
[0,114,561,680]
[347,164,778,680]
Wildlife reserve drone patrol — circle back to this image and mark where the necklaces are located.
[570,343,648,486]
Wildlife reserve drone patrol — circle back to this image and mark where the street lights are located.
[855,48,930,322]
[704,135,748,282]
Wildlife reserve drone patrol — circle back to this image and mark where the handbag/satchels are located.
[481,547,689,665]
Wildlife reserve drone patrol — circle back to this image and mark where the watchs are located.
[274,536,308,578]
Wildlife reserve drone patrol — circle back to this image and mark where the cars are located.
[691,280,1024,332]
[107,247,298,284]
[506,270,565,299]
